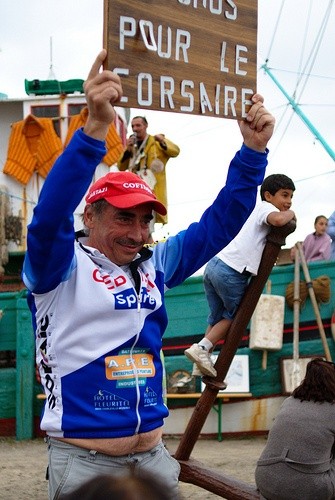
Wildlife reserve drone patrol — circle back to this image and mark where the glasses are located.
[314,357,335,370]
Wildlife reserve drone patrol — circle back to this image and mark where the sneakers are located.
[191,363,206,377]
[184,343,217,378]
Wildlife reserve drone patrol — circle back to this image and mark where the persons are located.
[70,470,180,500]
[183,173,295,389]
[255,357,335,500]
[117,115,180,245]
[22,49,275,500]
[327,211,335,259]
[290,215,332,262]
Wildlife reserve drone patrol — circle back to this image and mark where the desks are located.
[35,390,252,442]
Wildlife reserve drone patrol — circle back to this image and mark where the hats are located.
[85,171,168,217]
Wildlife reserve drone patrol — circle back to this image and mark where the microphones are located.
[129,132,137,146]
